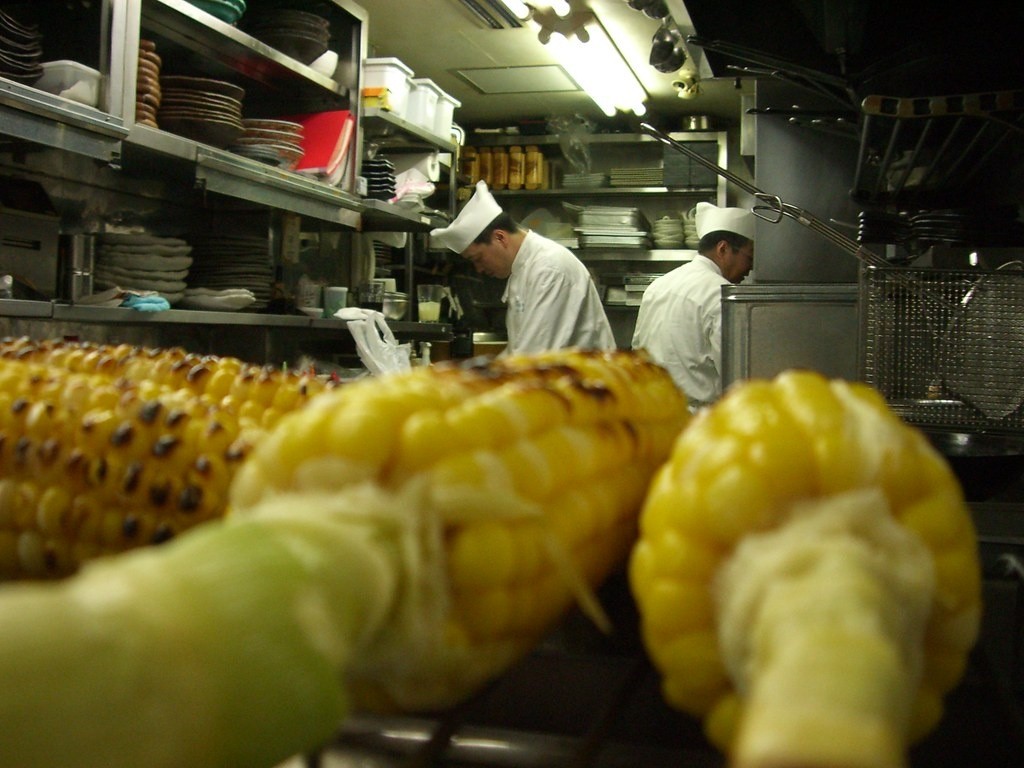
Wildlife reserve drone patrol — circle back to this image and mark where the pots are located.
[682,115,713,131]
[854,212,988,251]
[913,431,1024,502]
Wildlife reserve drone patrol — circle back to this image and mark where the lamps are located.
[496,0,649,117]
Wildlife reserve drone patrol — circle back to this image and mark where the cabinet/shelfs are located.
[0,0,458,337]
[460,129,728,315]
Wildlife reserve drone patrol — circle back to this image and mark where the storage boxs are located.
[361,55,462,141]
[33,59,103,107]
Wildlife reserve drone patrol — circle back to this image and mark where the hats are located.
[430,179,503,255]
[695,202,753,240]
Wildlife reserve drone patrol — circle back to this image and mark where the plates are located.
[361,159,397,201]
[398,193,425,214]
[93,236,271,311]
[684,220,704,249]
[0,0,44,87]
[187,0,247,24]
[609,167,664,187]
[156,74,305,169]
[298,304,325,318]
[653,216,684,249]
[561,172,608,187]
[368,238,392,267]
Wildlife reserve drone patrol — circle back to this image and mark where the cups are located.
[324,286,348,319]
[417,284,443,323]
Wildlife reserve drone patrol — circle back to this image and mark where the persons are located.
[430,180,618,357]
[631,203,755,416]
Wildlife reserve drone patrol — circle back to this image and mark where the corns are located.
[627,367,979,768]
[0,323,691,768]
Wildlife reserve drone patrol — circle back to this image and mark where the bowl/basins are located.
[253,10,332,67]
[382,291,410,321]
